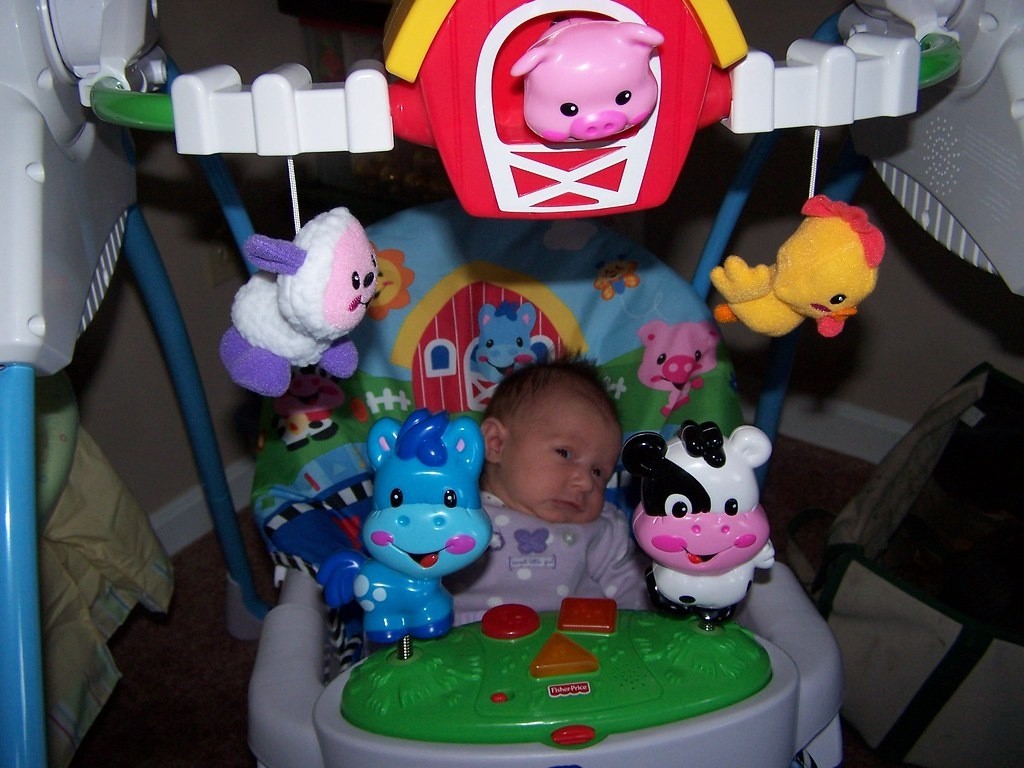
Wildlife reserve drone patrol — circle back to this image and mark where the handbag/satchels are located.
[777,361,1024,768]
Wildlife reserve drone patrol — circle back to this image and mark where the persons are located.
[441,348,652,624]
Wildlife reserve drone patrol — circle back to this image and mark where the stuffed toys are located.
[710,196,886,337]
[217,205,378,397]
